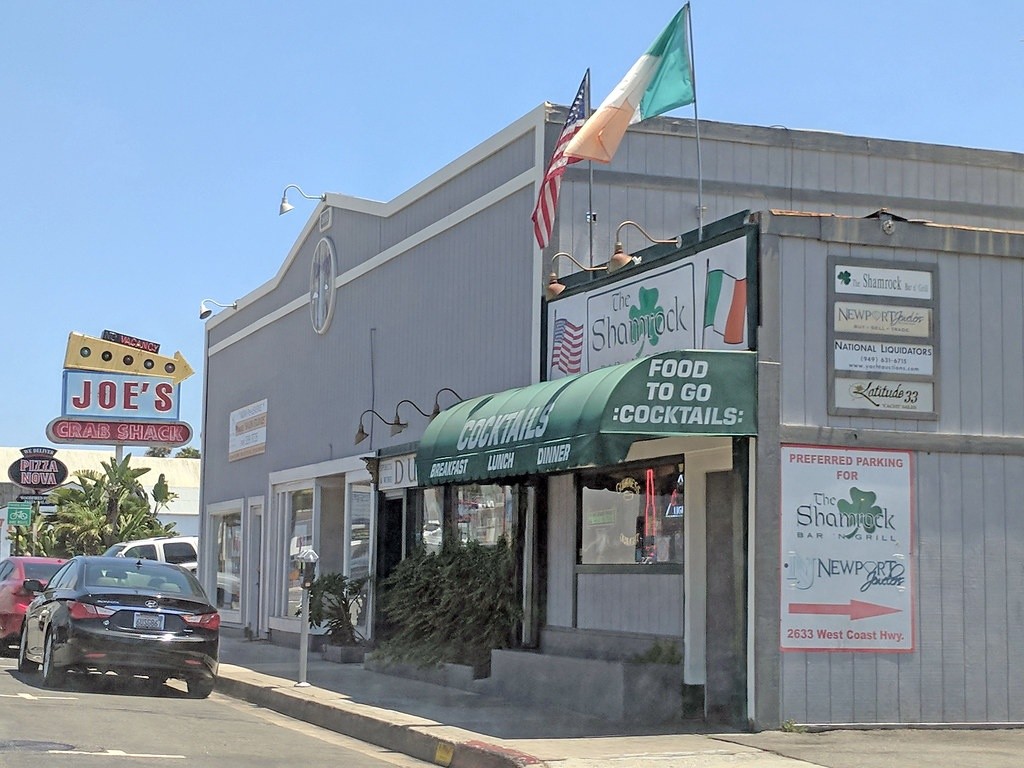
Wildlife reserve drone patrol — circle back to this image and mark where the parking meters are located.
[294,548,320,688]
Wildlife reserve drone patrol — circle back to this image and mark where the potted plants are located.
[295,571,375,664]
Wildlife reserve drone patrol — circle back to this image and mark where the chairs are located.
[96,576,117,587]
[160,583,181,592]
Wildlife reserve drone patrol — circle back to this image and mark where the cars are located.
[419,519,472,548]
[352,514,370,544]
[0,556,76,657]
[218,573,241,600]
[18,557,222,699]
[102,535,198,583]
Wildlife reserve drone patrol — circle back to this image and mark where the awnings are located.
[415,350,757,488]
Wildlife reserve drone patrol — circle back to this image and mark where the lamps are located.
[608,221,682,272]
[430,388,463,422]
[547,252,610,298]
[391,400,430,437]
[199,299,237,319]
[354,410,408,445]
[279,185,326,215]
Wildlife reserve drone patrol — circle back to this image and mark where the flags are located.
[563,4,693,163]
[530,76,587,249]
